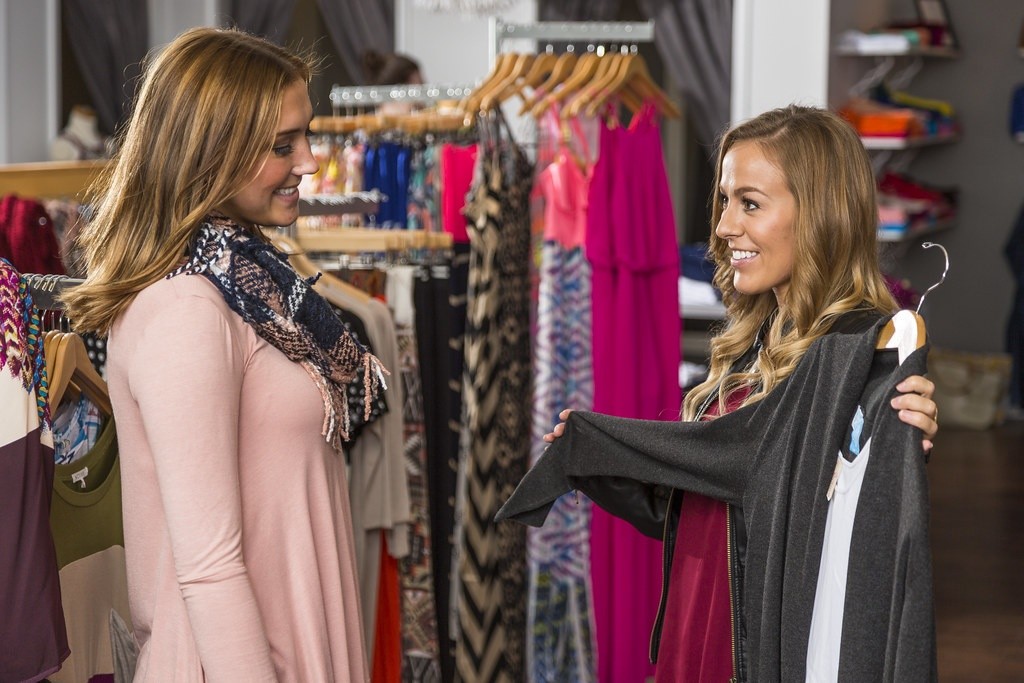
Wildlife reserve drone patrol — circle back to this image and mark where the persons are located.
[53,105,117,161]
[63,27,392,682]
[367,52,430,115]
[542,105,939,682]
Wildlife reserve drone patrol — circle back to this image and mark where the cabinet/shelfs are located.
[836,48,964,240]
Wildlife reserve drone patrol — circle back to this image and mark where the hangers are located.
[21,274,113,421]
[259,190,453,302]
[309,20,681,135]
[876,241,949,366]
